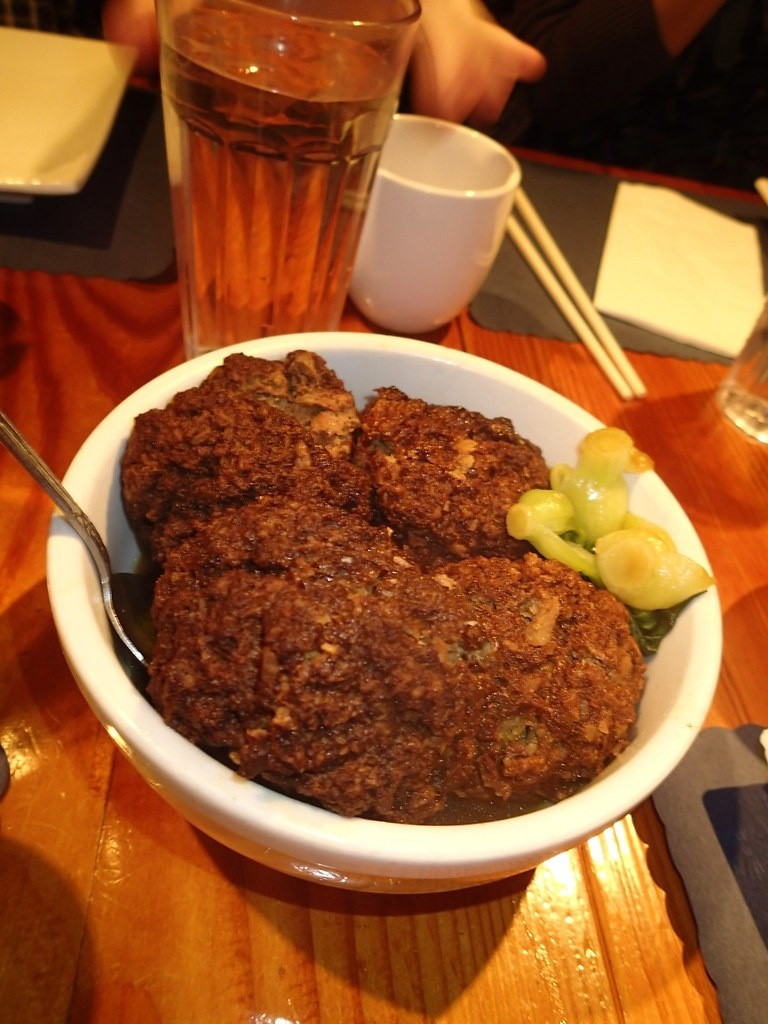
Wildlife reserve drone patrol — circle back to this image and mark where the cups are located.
[347,114,521,334]
[154,0,422,360]
[716,298,768,445]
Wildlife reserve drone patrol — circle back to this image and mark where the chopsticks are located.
[508,188,645,401]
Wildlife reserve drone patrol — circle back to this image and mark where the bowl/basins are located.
[46,331,725,897]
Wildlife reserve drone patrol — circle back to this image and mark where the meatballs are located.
[122,348,647,822]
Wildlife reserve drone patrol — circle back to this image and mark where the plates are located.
[0,25,139,196]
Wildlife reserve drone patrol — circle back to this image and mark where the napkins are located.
[592,189,764,359]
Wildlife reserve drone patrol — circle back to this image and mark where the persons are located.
[104,0,768,199]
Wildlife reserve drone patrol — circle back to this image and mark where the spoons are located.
[0,412,158,671]
